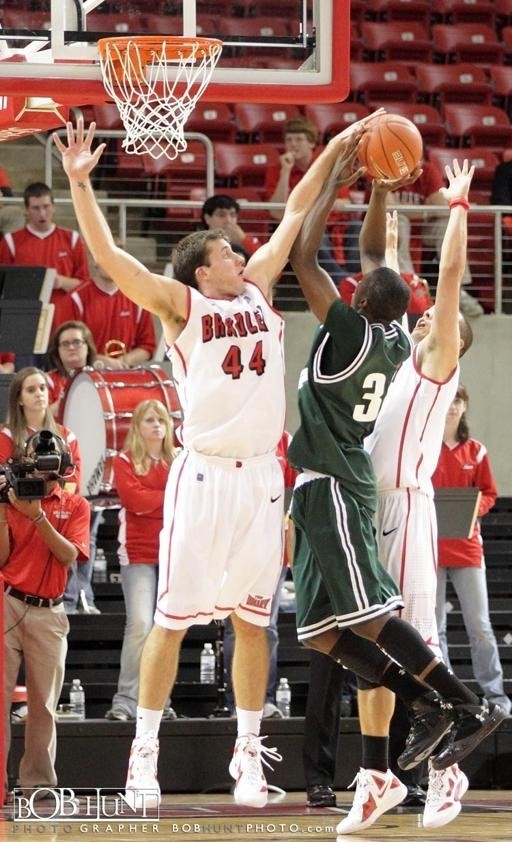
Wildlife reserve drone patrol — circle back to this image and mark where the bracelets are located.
[449,198,471,210]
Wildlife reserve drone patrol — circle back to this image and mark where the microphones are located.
[48,465,76,480]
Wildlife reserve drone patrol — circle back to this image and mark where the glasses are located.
[58,339,84,347]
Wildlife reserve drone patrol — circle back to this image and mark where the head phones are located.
[22,430,70,474]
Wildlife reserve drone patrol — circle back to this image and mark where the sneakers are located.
[423,756,470,829]
[397,703,458,771]
[432,698,507,771]
[78,598,101,615]
[228,738,268,809]
[306,785,336,807]
[384,784,430,813]
[159,705,176,720]
[337,766,408,834]
[125,739,162,811]
[105,703,131,720]
[491,697,511,716]
[22,787,65,800]
[262,702,286,717]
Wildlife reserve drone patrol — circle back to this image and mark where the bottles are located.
[93,546,107,583]
[200,641,217,686]
[69,676,86,720]
[275,676,292,719]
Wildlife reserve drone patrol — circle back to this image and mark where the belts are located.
[3,588,65,606]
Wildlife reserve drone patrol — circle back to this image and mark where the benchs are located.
[0,0,512,279]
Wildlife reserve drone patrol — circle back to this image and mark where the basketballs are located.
[358,113,423,179]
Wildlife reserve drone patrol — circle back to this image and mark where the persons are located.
[1,429,91,806]
[222,534,288,719]
[105,399,184,722]
[201,194,260,267]
[284,493,427,808]
[431,383,512,717]
[51,105,387,810]
[335,157,474,835]
[285,123,508,770]
[0,182,89,344]
[275,428,299,488]
[47,320,101,615]
[55,257,156,371]
[0,365,82,721]
[263,114,362,286]
[363,159,484,317]
